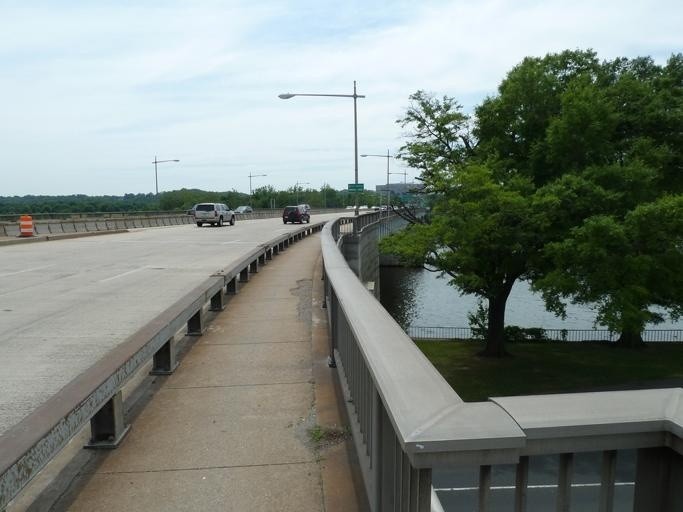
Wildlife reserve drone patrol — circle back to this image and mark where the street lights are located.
[296,181,310,205]
[389,170,408,188]
[278,80,366,216]
[248,170,267,195]
[360,149,393,209]
[152,155,180,194]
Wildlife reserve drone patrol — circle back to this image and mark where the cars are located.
[233,206,254,213]
[345,206,398,210]
[301,204,311,211]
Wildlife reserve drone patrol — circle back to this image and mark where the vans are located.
[195,203,235,227]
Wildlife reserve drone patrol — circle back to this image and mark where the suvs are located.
[283,206,310,224]
